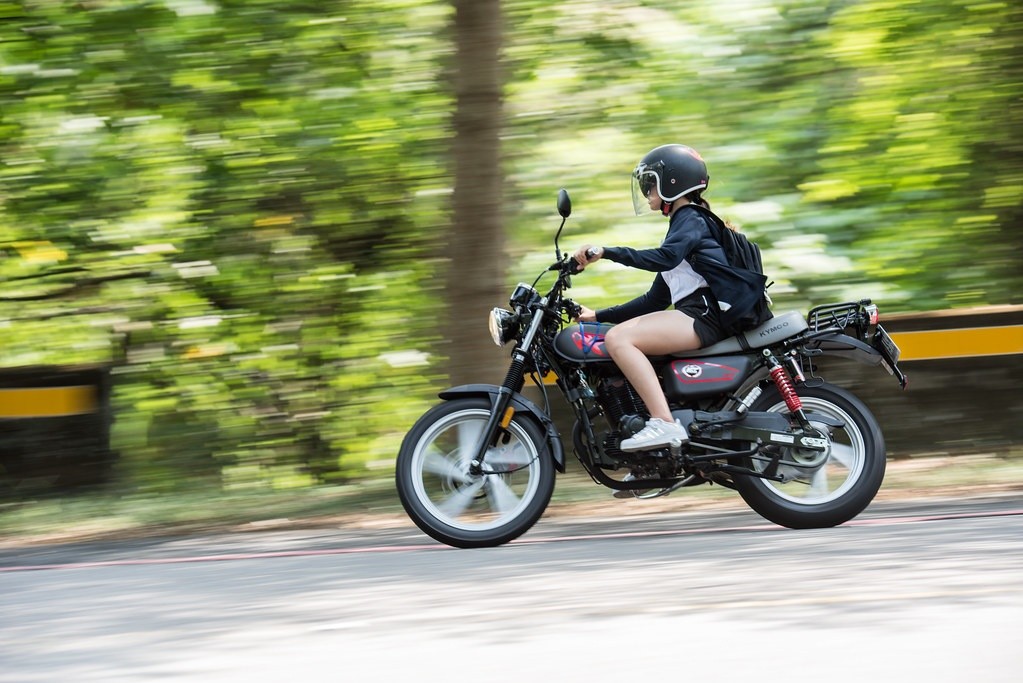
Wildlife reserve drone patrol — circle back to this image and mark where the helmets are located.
[631,144,709,216]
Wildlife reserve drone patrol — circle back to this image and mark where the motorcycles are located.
[395,187,911,548]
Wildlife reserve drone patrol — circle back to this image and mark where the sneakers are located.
[621,417,689,453]
[613,471,660,499]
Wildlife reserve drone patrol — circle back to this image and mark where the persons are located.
[574,144,740,499]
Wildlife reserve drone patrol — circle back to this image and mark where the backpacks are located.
[669,203,774,331]
